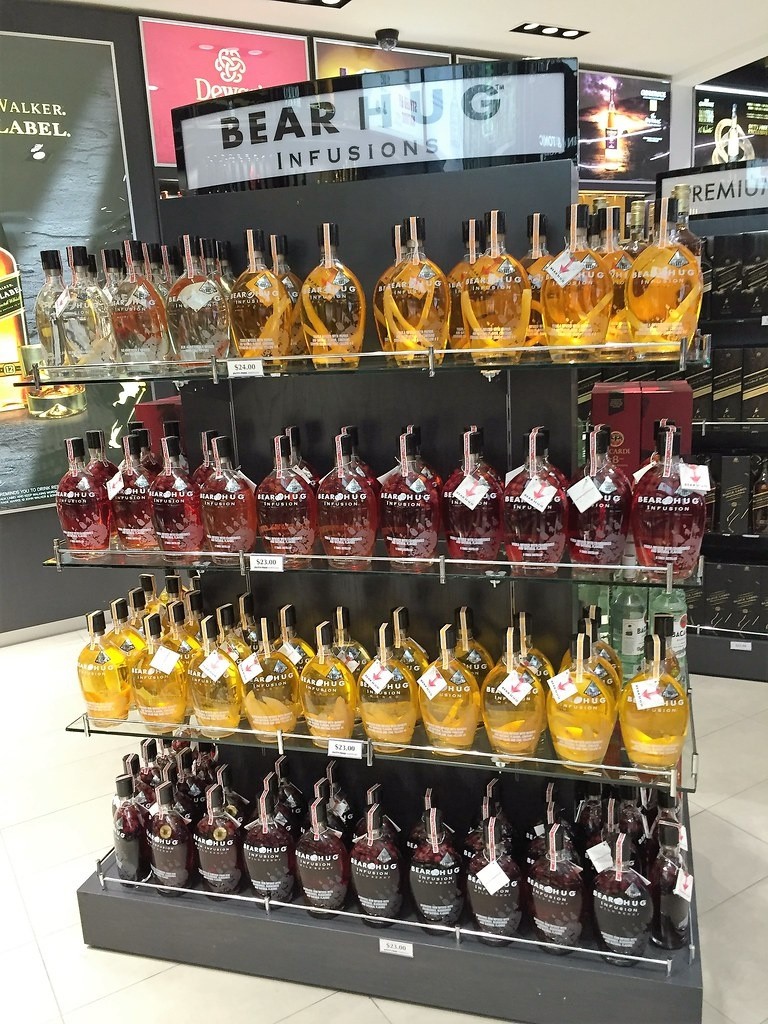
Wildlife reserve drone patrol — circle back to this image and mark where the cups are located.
[18,344,88,418]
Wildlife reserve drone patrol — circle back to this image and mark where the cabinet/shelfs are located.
[14,57,703,1024]
[652,158,768,682]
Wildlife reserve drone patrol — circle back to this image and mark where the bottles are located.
[372,197,704,357]
[36,222,364,369]
[55,420,707,967]
[0,249,32,413]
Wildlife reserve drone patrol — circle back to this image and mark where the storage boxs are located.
[593,381,694,483]
[686,231,768,633]
